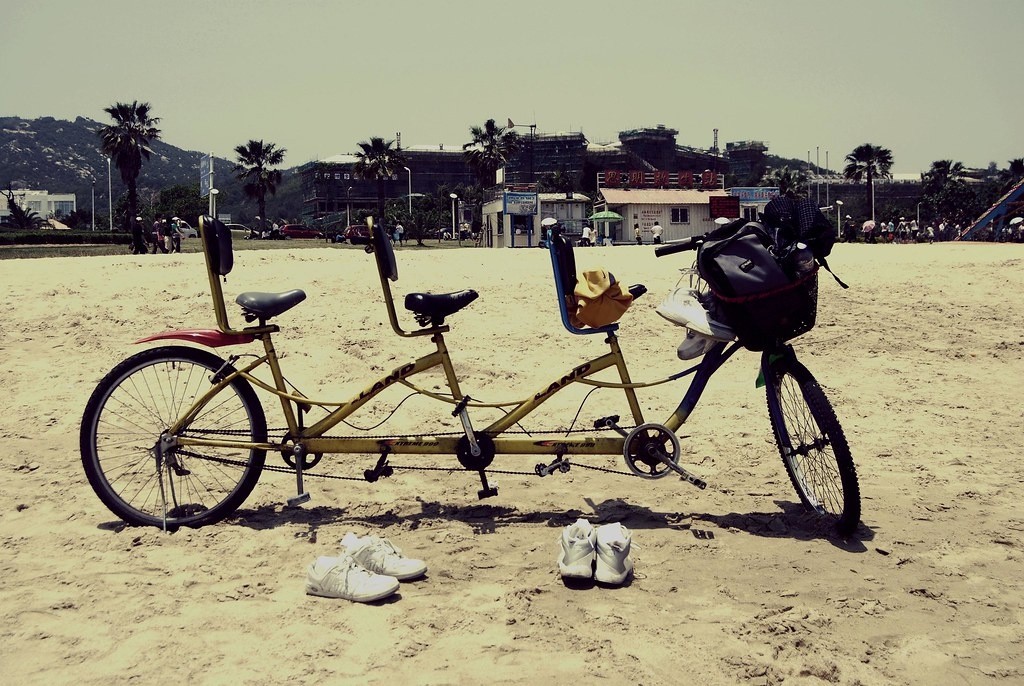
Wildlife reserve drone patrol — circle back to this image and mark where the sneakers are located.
[677,290,718,360]
[304,555,400,602]
[655,286,736,342]
[593,522,633,584]
[557,519,596,578]
[341,532,427,579]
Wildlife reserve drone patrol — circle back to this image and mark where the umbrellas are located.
[1009,217,1023,224]
[588,211,623,222]
[863,220,875,232]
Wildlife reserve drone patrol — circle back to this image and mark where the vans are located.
[158,220,198,238]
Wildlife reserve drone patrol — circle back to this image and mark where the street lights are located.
[106,158,113,231]
[404,167,412,217]
[347,186,352,226]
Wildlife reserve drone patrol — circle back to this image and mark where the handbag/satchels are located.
[696,221,807,337]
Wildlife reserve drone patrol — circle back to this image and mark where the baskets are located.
[711,260,822,352]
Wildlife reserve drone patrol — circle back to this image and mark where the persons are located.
[651,221,664,244]
[390,220,404,247]
[244,222,289,240]
[443,230,451,240]
[582,225,597,247]
[634,223,643,245]
[332,232,348,243]
[841,214,1024,244]
[129,213,185,255]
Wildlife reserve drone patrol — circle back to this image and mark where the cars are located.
[279,224,324,239]
[344,225,392,240]
[224,224,260,238]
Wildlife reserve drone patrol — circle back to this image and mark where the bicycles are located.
[79,187,862,538]
